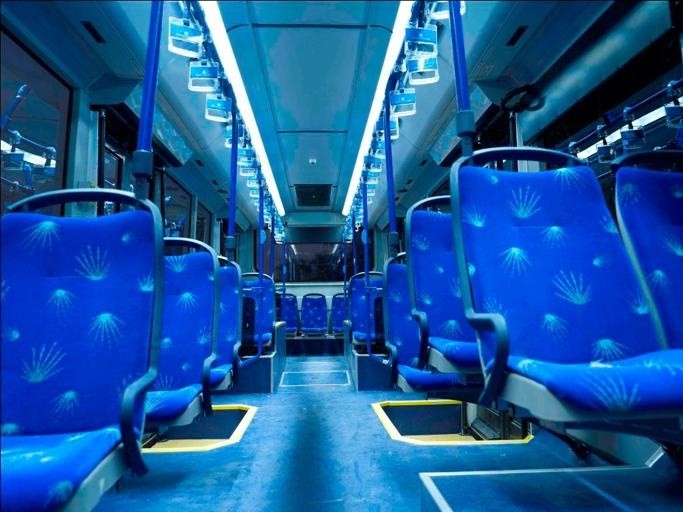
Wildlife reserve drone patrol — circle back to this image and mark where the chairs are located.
[0,146,683,512]
